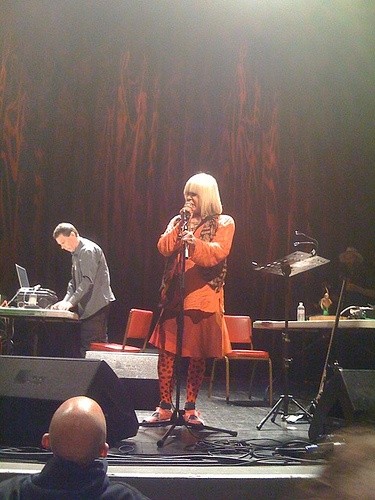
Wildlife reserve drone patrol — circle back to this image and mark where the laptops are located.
[15,264,56,294]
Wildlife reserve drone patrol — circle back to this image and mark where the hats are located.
[339,246,363,264]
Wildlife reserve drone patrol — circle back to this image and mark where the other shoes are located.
[141,406,204,429]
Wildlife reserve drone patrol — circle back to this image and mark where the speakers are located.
[307,369,375,440]
[0,350,161,446]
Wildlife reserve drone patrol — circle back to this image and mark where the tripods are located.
[252,250,331,430]
[140,209,237,447]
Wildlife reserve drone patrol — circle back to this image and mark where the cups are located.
[29,294,37,307]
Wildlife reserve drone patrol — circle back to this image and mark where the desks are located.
[252,320,375,430]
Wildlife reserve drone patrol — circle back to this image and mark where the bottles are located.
[296,302,305,321]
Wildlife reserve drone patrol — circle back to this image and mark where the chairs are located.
[89,308,153,352]
[207,315,273,407]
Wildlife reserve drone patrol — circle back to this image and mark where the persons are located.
[52,223,116,356]
[0,396,151,500]
[321,246,374,318]
[142,173,235,427]
